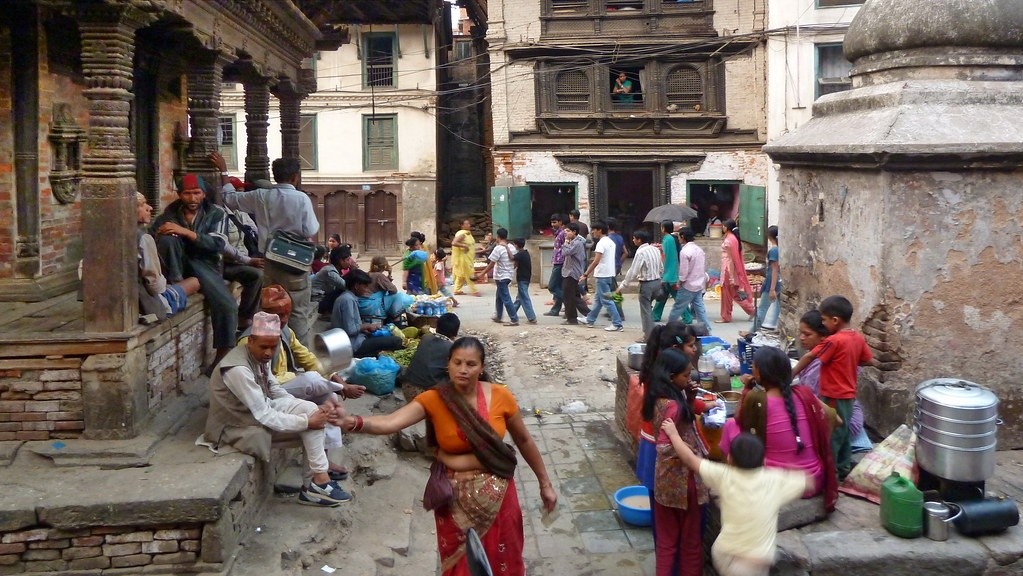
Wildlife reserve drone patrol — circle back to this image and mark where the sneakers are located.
[297,485,341,507]
[444,291,780,343]
[327,462,347,480]
[305,476,352,502]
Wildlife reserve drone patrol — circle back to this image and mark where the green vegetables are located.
[388,340,419,367]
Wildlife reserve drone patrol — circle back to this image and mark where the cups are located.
[923,501,950,541]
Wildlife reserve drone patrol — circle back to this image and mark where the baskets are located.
[404,309,448,329]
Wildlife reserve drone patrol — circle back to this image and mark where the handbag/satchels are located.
[242,226,259,253]
[264,228,318,275]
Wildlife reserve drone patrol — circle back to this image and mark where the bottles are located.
[880,470,924,537]
[416,303,446,315]
[698,354,731,393]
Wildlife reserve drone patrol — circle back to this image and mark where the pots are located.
[912,378,1004,481]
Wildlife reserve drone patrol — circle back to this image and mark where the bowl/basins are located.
[314,327,351,373]
[628,344,647,370]
[614,485,652,526]
[716,391,741,415]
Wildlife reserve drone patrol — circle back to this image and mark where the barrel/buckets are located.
[709,223,722,239]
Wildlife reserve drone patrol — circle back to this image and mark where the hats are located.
[252,311,282,337]
[176,174,206,193]
[262,284,292,314]
[135,191,148,204]
[230,176,252,188]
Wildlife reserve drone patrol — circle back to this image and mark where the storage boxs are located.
[737,337,763,376]
[473,271,488,284]
[694,335,732,354]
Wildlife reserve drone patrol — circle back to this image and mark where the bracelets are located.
[349,413,364,434]
[582,273,588,277]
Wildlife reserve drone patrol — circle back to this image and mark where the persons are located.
[577,223,624,333]
[737,225,780,340]
[499,238,539,325]
[659,416,815,576]
[560,222,593,325]
[614,229,665,344]
[130,148,461,362]
[717,346,843,501]
[320,335,558,576]
[651,218,693,325]
[478,232,498,264]
[798,312,865,443]
[714,217,757,324]
[666,224,713,337]
[234,280,367,481]
[603,217,628,322]
[788,295,874,485]
[568,209,593,304]
[470,228,519,327]
[451,219,483,298]
[640,346,721,576]
[636,322,697,492]
[543,214,569,316]
[702,204,722,237]
[201,311,354,508]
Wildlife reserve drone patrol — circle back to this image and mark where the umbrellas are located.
[642,201,698,222]
